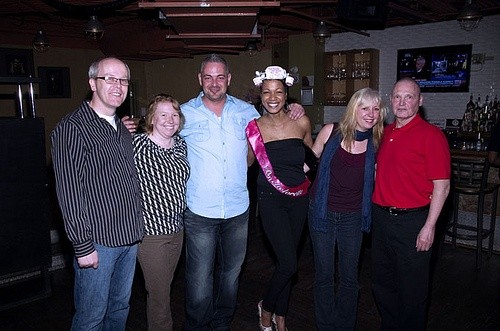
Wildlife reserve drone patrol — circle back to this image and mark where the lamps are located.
[83,15,105,45]
[32,30,52,53]
[312,21,331,46]
[456,0,483,32]
[245,41,258,57]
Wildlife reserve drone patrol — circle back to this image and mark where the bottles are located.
[352,61,361,79]
[447,131,486,151]
[482,95,500,131]
[361,60,371,79]
[326,93,346,103]
[463,95,484,131]
[327,62,346,78]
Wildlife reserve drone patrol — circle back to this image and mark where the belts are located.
[378,206,424,215]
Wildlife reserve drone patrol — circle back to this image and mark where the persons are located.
[131,94,190,331]
[122,55,304,331]
[305,88,383,331]
[51,57,144,331]
[411,56,431,81]
[248,66,312,331]
[369,77,451,331]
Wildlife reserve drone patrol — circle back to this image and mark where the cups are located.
[313,111,324,132]
[126,115,141,130]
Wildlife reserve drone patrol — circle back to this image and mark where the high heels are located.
[258,300,273,331]
[272,313,288,331]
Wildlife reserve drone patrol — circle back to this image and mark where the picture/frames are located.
[37,66,71,99]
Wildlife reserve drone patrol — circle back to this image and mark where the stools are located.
[433,156,500,272]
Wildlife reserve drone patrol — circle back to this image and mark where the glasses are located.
[94,76,130,86]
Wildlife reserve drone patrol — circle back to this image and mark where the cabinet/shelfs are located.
[321,48,379,106]
[0,117,52,310]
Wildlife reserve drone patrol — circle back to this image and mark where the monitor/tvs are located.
[396,44,473,93]
